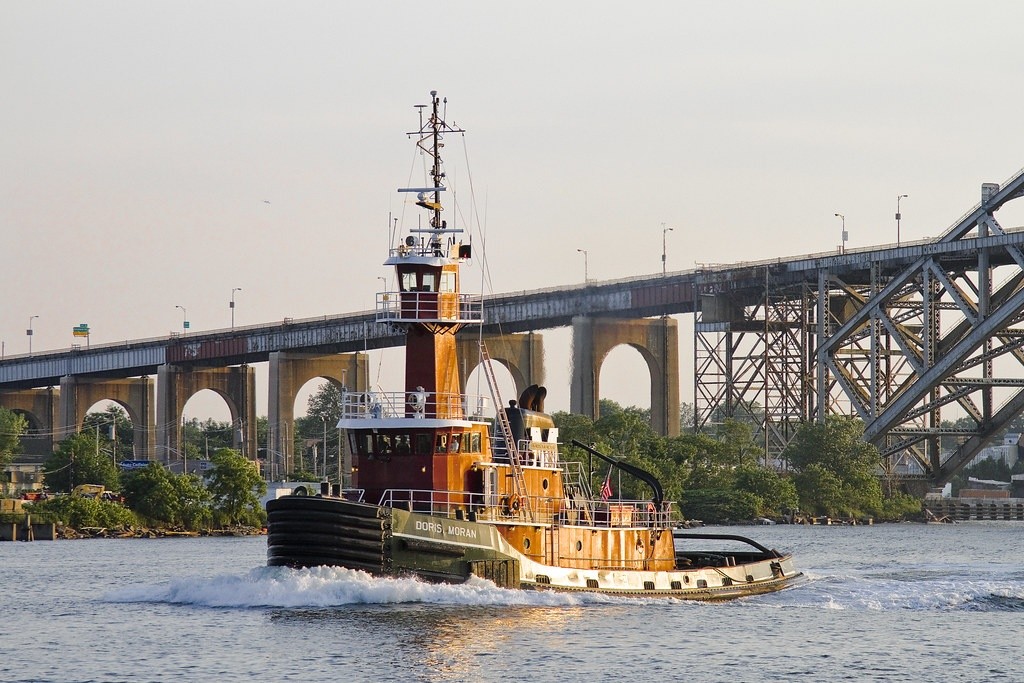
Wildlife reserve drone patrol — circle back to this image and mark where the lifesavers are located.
[293,485,309,496]
[508,493,522,513]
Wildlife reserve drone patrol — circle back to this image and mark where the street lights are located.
[662,228,673,273]
[576,249,587,283]
[834,213,848,254]
[230,287,242,327]
[176,305,189,336]
[896,195,908,248]
[377,277,386,310]
[27,315,40,353]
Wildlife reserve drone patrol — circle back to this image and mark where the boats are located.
[264,89,812,605]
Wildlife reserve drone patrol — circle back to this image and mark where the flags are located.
[600,471,612,501]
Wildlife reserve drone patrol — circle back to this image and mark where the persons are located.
[397,436,409,455]
[378,435,389,452]
[451,439,459,452]
[417,437,431,452]
